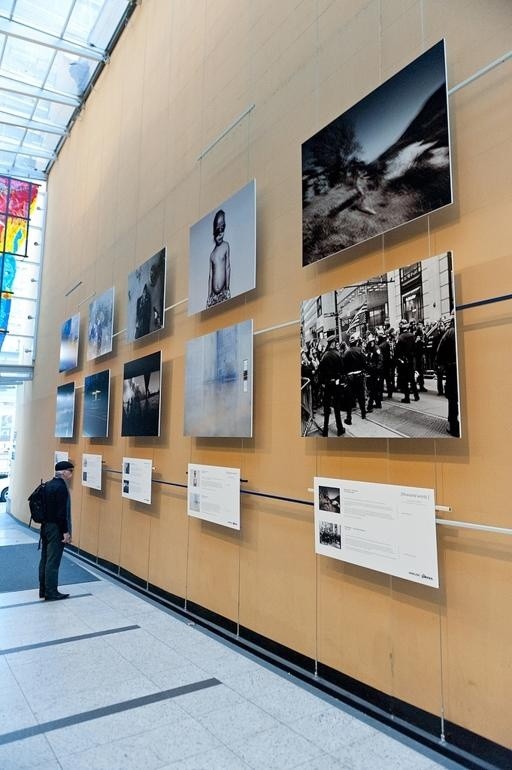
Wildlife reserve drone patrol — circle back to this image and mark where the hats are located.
[54,461,74,471]
[311,314,454,358]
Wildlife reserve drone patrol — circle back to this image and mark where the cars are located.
[0,459,12,502]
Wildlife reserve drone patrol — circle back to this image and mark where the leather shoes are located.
[420,388,427,392]
[319,430,328,437]
[38,589,70,602]
[344,419,351,425]
[373,405,382,408]
[337,428,345,436]
[401,399,411,403]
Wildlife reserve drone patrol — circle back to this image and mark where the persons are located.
[152,306,159,324]
[206,209,230,307]
[34,461,72,601]
[303,307,458,436]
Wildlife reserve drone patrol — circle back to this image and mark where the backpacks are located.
[28,481,64,523]
